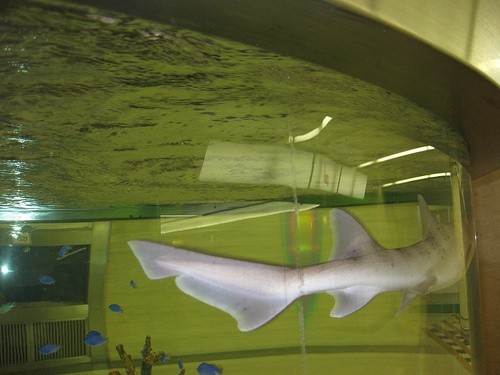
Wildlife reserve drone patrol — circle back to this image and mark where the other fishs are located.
[21,225,38,232]
[178,359,183,369]
[58,245,72,256]
[39,344,61,354]
[83,330,109,347]
[197,363,223,375]
[129,280,137,288]
[23,247,31,253]
[161,352,169,364]
[0,302,15,314]
[109,304,123,313]
[127,190,477,332]
[38,275,54,285]
